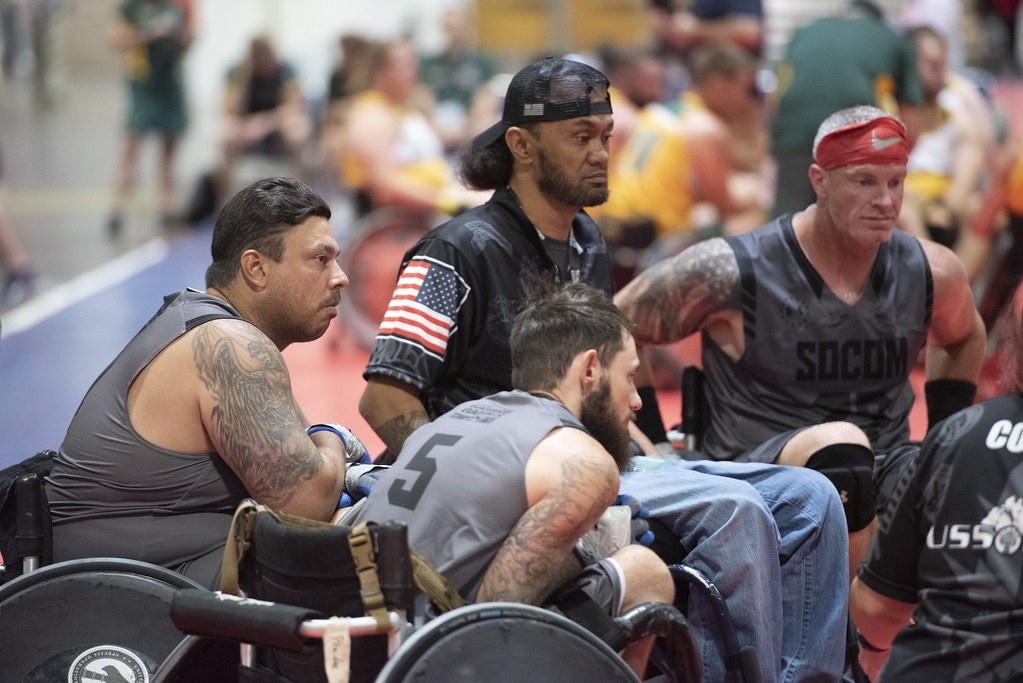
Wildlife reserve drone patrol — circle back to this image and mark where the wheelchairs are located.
[599,215,717,390]
[0,450,265,683]
[148,501,705,683]
[334,191,465,360]
[329,443,870,683]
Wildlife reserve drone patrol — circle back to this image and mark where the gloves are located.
[575,491,655,564]
[340,459,392,496]
[305,421,375,506]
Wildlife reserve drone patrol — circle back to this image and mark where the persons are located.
[613,105,986,683]
[43,176,390,596]
[360,55,850,683]
[851,284,1023,683]
[350,282,674,683]
[216,0,1015,279]
[104,0,195,237]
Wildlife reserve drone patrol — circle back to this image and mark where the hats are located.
[471,56,612,144]
